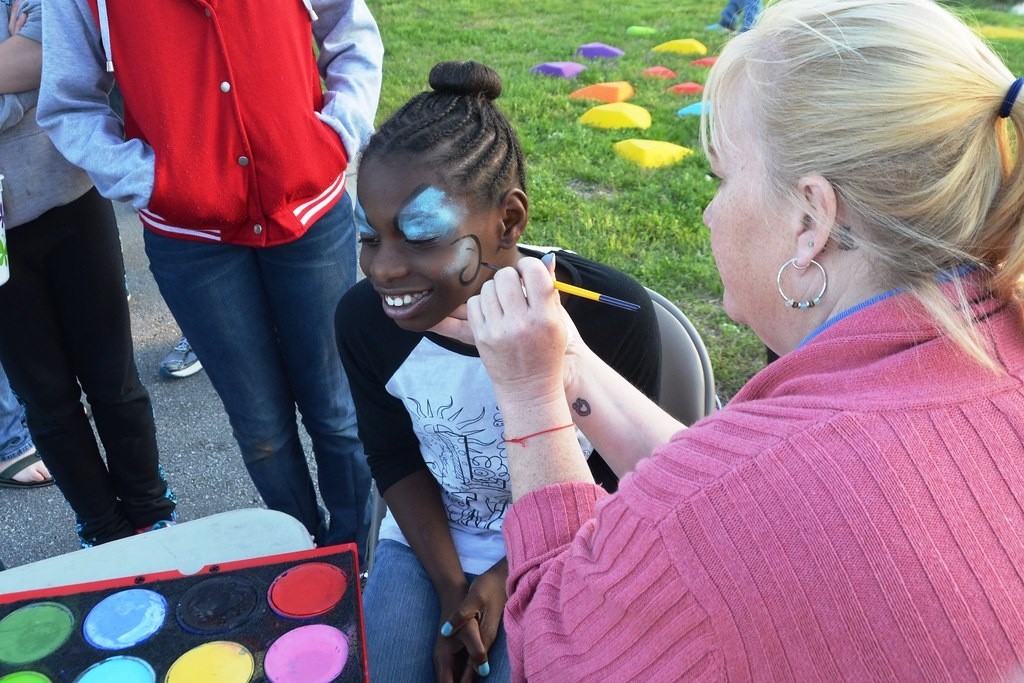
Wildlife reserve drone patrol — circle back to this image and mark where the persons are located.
[0,0,381,590]
[334,1,1023,683]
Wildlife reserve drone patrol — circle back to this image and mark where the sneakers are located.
[159,336,203,379]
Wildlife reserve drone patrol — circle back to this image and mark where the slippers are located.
[0,451,56,488]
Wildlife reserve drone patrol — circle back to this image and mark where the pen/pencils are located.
[480,261,640,312]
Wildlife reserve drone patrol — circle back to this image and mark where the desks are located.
[0,508,317,594]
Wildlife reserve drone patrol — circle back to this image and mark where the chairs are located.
[367,289,716,589]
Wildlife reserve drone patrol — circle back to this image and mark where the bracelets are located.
[502,422,578,444]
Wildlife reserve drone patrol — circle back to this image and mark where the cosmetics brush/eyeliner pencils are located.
[480,262,641,312]
